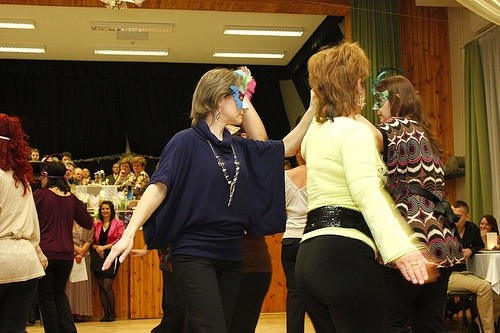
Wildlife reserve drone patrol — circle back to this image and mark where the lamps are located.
[0,17,304,59]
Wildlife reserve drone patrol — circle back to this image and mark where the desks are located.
[471,253,500,295]
[75,185,117,201]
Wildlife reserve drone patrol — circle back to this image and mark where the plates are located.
[478,249,500,253]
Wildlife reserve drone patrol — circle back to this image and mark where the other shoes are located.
[100,314,115,322]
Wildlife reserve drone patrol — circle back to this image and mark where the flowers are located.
[234,66,257,101]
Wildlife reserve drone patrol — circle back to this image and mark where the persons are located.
[281,145,307,333]
[447,201,495,333]
[294,41,435,333]
[29,153,93,333]
[479,215,500,251]
[130,243,186,333]
[223,92,292,333]
[29,148,151,257]
[0,113,49,333]
[101,66,320,333]
[76,201,126,322]
[355,70,465,333]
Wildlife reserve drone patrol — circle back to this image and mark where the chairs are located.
[441,290,472,333]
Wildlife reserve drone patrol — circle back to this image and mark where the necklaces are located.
[103,222,110,228]
[207,139,240,207]
